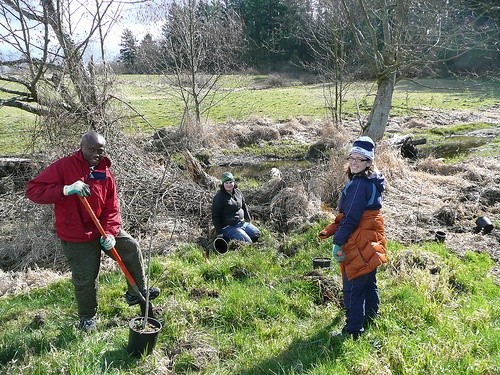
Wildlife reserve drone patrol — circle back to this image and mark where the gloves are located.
[332,244,347,262]
[99,233,117,251]
[63,180,91,198]
[217,234,224,239]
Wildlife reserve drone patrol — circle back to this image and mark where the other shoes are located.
[329,331,342,337]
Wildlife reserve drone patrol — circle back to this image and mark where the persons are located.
[212,172,260,243]
[317,137,388,341]
[25,131,160,330]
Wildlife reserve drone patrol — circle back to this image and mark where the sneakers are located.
[79,318,97,334]
[124,287,160,306]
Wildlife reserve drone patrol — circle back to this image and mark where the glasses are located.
[346,155,370,163]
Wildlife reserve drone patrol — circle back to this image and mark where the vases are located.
[127,316,162,358]
[205,238,228,255]
[311,258,331,270]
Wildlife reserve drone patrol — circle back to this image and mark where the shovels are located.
[76,192,154,319]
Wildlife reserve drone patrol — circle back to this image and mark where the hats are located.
[349,136,375,162]
[221,172,235,184]
